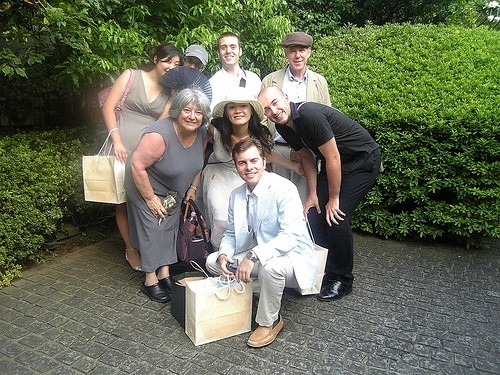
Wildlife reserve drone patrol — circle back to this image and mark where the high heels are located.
[126,248,143,272]
[158,275,177,294]
[142,281,170,302]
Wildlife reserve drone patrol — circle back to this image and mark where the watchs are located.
[246,251,258,262]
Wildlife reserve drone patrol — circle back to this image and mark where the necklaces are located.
[173,122,197,149]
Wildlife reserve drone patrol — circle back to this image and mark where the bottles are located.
[188,212,196,224]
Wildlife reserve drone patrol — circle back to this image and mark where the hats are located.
[280,32,313,46]
[212,92,264,121]
[185,44,208,66]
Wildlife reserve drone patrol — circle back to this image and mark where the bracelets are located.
[189,185,196,190]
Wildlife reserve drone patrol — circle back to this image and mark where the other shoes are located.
[248,314,284,348]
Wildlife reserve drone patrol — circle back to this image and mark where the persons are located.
[102,43,180,272]
[180,45,208,73]
[123,89,211,303]
[205,32,261,129]
[199,88,306,254]
[258,86,381,302]
[261,31,331,205]
[205,138,318,347]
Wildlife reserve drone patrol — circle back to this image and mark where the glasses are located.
[186,58,204,69]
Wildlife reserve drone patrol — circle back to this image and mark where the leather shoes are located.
[318,280,351,301]
[320,276,334,288]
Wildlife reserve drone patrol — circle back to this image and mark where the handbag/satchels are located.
[185,274,253,346]
[97,68,134,120]
[177,199,208,263]
[184,201,208,237]
[82,128,127,204]
[170,260,215,329]
[294,216,329,295]
